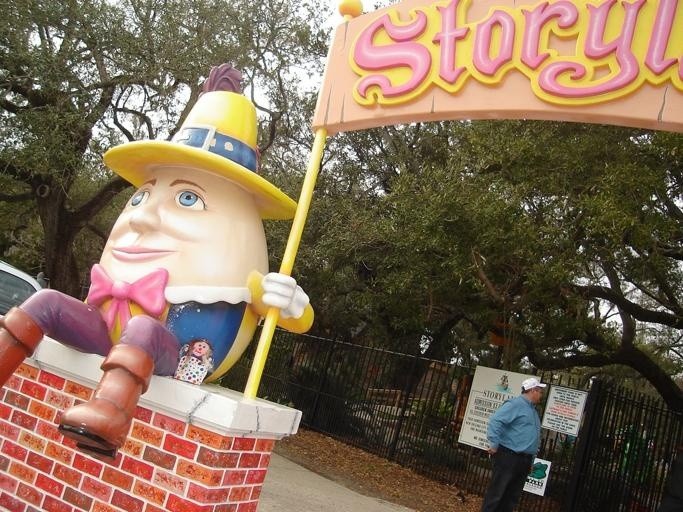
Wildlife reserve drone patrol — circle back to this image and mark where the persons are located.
[481,377,548,511]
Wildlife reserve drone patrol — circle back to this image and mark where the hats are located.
[520,378,547,392]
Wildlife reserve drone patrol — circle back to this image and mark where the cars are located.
[0,257,45,318]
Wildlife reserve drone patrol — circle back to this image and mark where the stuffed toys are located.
[173,338,214,386]
